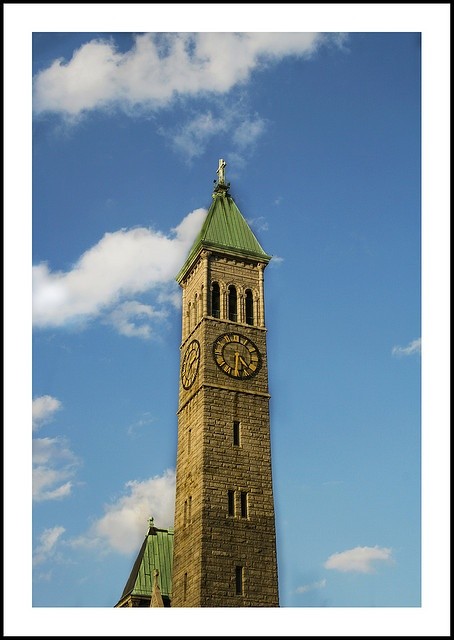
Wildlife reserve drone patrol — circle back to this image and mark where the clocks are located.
[212,332,262,380]
[181,339,200,389]
